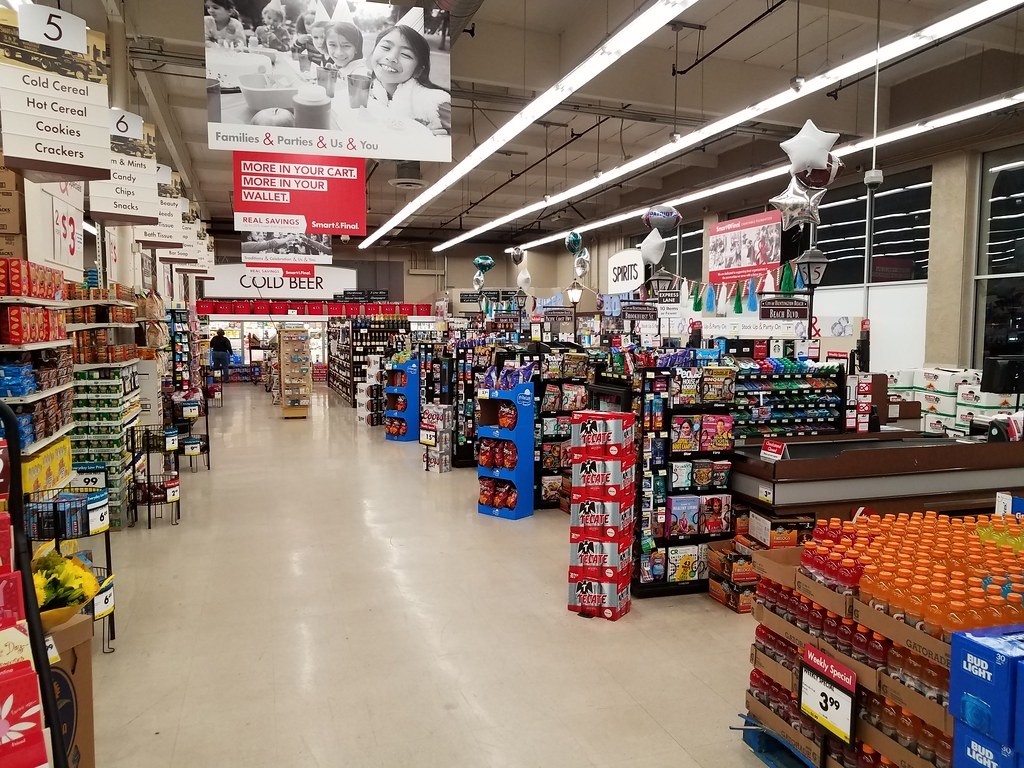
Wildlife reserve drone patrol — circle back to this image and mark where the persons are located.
[204,0,451,139]
[680,419,696,449]
[550,393,561,410]
[723,378,734,399]
[241,230,332,255]
[675,375,681,392]
[568,387,585,409]
[210,329,233,383]
[471,313,485,328]
[677,512,688,535]
[705,498,730,533]
[708,419,732,450]
[709,224,779,269]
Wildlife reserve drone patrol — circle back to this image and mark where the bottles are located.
[328,313,410,407]
[745,508,1024,768]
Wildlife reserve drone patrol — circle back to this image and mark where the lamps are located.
[356,0,1024,254]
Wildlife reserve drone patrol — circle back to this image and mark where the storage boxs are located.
[952,716,1017,768]
[705,517,952,768]
[888,368,1024,438]
[950,623,1024,747]
[196,300,433,316]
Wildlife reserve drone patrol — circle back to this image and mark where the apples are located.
[250,108,294,127]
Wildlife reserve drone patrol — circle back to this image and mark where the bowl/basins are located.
[237,73,300,110]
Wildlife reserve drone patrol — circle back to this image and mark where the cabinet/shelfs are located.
[208,321,244,365]
[0,258,224,768]
[247,295,888,601]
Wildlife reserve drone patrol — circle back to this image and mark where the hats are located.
[331,0,354,24]
[394,6,426,38]
[313,0,332,23]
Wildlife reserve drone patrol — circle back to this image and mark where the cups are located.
[206,78,222,123]
[316,67,339,98]
[347,74,372,109]
[298,54,312,72]
